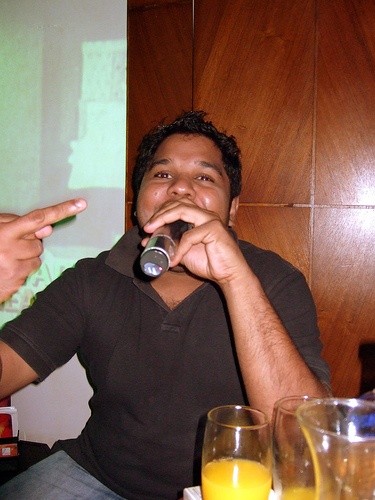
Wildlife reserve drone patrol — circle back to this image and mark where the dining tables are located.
[183,480,278,500]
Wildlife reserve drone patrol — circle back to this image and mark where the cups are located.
[272,395,375,499]
[201,404,272,500]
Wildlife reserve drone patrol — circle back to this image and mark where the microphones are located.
[140,219,193,277]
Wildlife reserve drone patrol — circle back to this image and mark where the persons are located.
[0,109,331,500]
